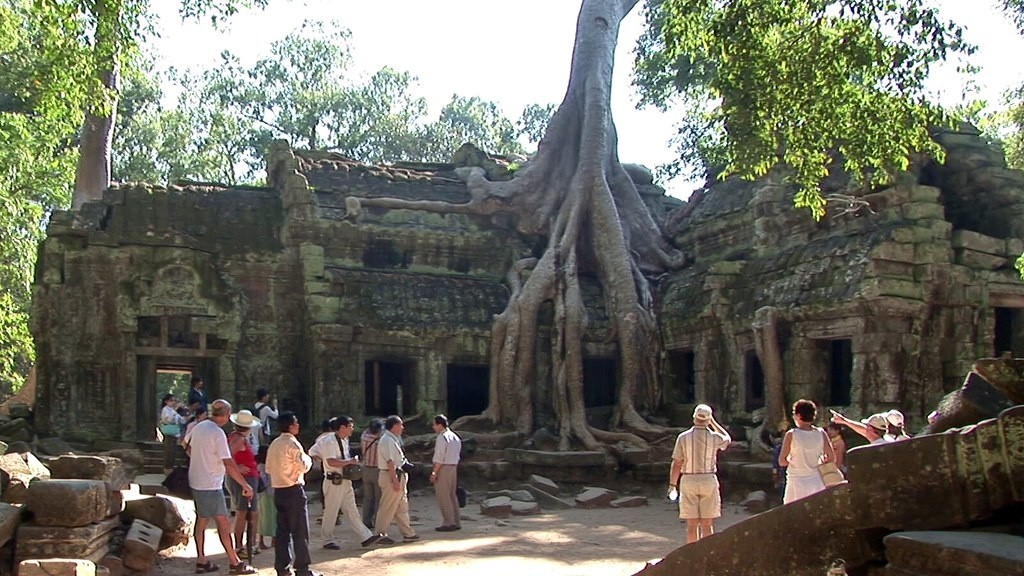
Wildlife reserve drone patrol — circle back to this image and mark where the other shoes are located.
[376,537,394,544]
[236,547,255,559]
[361,532,383,547]
[403,535,421,542]
[252,544,261,554]
[435,525,461,531]
[164,467,173,476]
[323,542,340,550]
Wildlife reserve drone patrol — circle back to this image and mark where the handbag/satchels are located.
[457,486,466,508]
[818,461,844,487]
[343,464,363,480]
[159,422,180,436]
[161,465,193,501]
[257,495,277,537]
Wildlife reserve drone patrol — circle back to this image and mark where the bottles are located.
[669,487,678,500]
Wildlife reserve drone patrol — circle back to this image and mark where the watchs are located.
[431,471,436,476]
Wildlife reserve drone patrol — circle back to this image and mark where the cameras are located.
[401,462,414,473]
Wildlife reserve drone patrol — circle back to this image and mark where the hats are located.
[692,404,712,421]
[861,414,888,430]
[229,409,261,428]
[882,409,904,427]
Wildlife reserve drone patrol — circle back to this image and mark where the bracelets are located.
[668,482,678,488]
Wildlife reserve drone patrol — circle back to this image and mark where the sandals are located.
[195,560,220,574]
[228,561,258,575]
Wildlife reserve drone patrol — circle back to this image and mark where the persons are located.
[771,426,792,506]
[189,375,207,413]
[362,419,385,529]
[667,404,732,544]
[253,388,279,446]
[183,406,208,469]
[777,399,836,505]
[306,415,383,550]
[429,413,463,532]
[180,398,260,575]
[159,393,192,478]
[256,444,277,549]
[826,559,848,576]
[827,422,846,468]
[223,409,262,560]
[265,410,323,576]
[828,409,911,444]
[372,416,422,545]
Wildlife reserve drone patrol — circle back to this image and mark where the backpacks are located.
[251,403,269,432]
